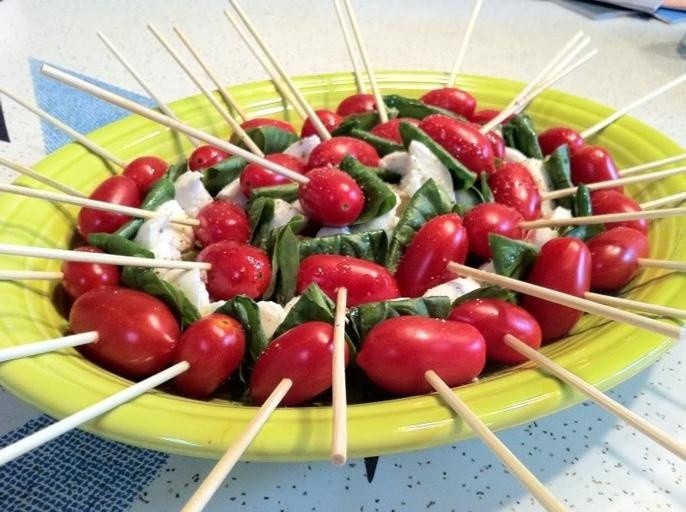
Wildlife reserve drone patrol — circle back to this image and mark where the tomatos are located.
[63,87,649,406]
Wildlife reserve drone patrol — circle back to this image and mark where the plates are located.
[1,70,686,463]
